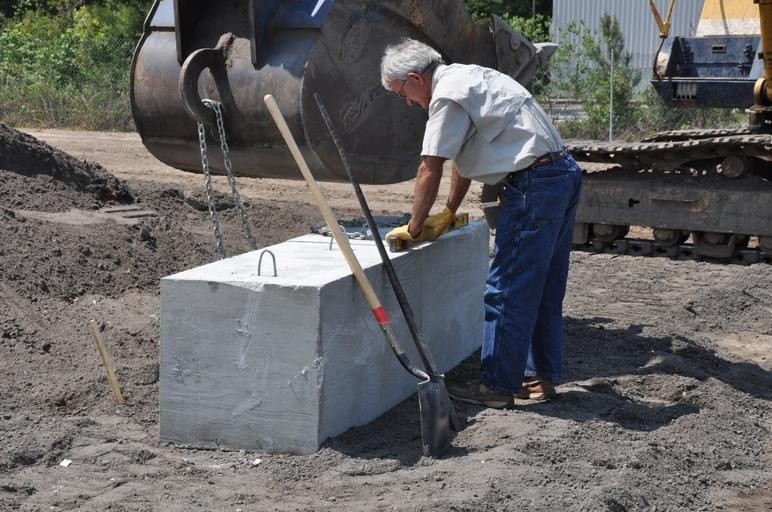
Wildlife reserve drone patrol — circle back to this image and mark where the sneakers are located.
[514,378,558,401]
[446,378,515,411]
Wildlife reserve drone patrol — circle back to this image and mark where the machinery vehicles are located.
[131,0,771,263]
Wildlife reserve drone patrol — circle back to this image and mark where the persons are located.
[381,34,582,411]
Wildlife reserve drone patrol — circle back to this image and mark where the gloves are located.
[385,205,459,249]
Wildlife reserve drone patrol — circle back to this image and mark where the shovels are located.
[264,92,449,456]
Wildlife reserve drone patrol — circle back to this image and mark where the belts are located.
[533,145,570,168]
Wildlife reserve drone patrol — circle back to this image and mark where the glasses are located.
[396,78,408,100]
